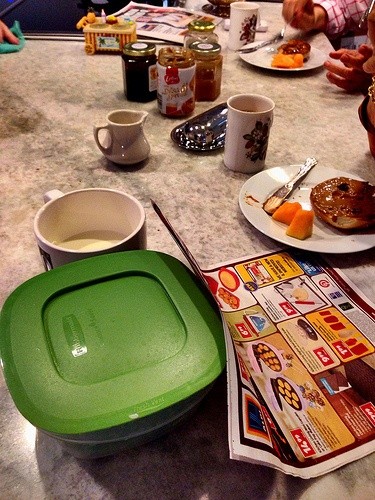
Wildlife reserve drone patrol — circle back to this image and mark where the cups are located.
[223,93,275,172]
[34,186,147,272]
[229,1,258,49]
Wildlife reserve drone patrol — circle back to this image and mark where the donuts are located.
[311,178,375,229]
[278,41,310,59]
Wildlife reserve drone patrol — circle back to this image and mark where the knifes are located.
[265,158,317,212]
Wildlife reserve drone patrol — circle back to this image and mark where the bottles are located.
[121,20,223,117]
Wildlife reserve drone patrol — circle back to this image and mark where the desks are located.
[0,0,375,500]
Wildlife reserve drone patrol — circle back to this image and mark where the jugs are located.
[94,110,150,165]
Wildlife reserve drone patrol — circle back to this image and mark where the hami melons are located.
[271,201,314,239]
[271,54,304,69]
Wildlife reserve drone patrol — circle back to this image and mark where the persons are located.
[281,0,375,157]
[0,19,20,46]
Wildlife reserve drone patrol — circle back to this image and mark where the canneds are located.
[121,21,223,118]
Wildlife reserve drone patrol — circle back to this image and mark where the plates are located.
[170,102,227,151]
[239,165,375,254]
[238,40,327,70]
